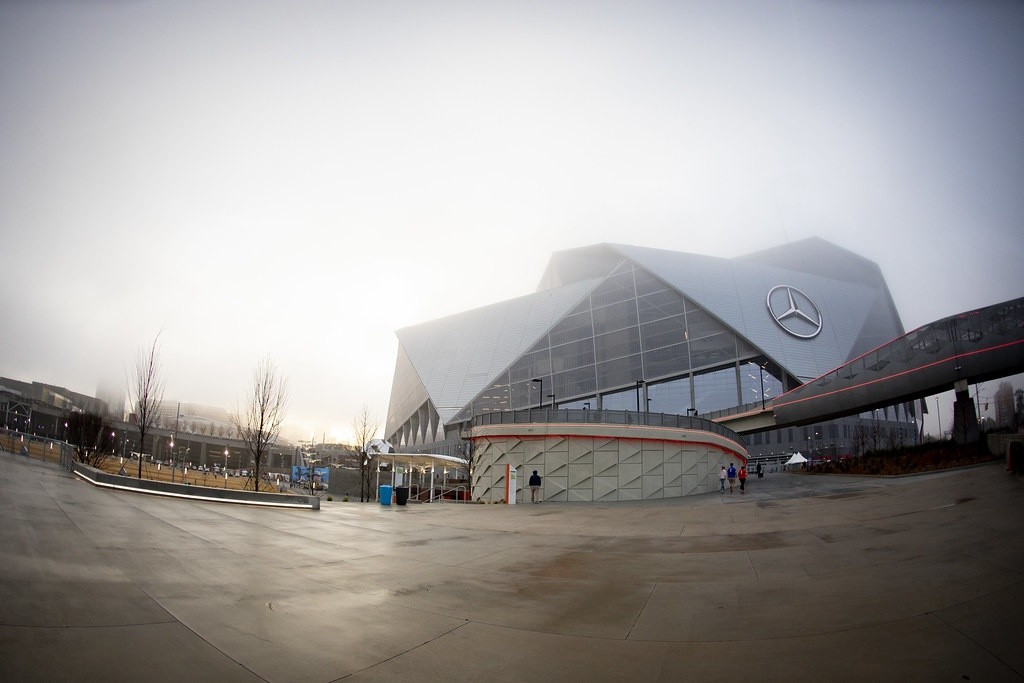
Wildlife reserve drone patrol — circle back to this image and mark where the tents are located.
[784,452,808,471]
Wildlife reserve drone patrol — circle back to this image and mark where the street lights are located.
[224,451,229,489]
[111,432,115,456]
[170,443,174,483]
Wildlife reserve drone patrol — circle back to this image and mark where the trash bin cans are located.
[396,487,409,505]
[1008,441,1023,469]
[379,485,393,505]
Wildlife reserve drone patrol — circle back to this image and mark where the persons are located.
[719,462,747,493]
[756,462,762,479]
[529,470,541,504]
